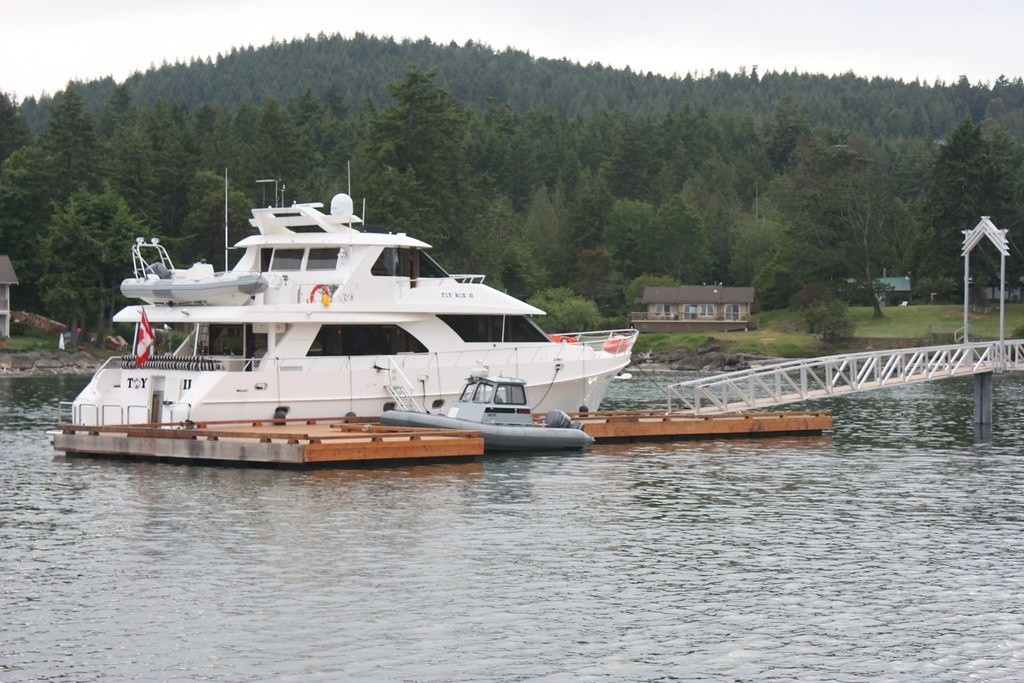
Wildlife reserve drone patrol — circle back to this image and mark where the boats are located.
[120,236,270,308]
[378,362,600,454]
[44,159,656,445]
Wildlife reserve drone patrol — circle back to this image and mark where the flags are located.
[137,310,154,365]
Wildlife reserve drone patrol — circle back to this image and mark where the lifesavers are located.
[310,286,329,304]
